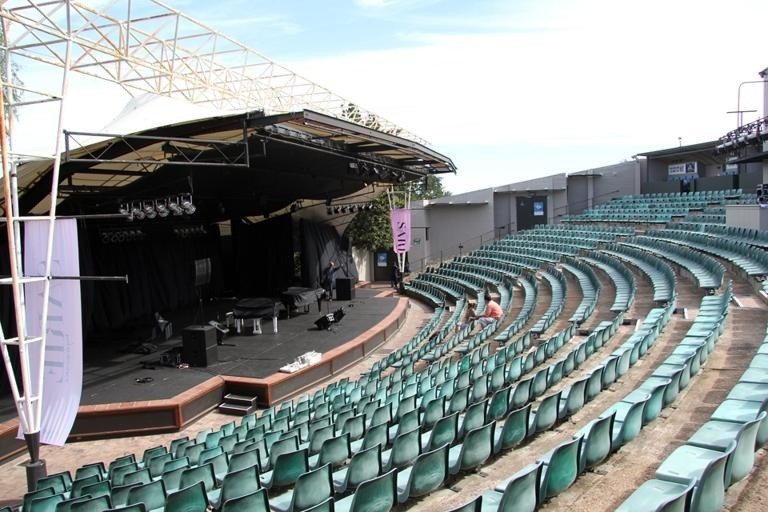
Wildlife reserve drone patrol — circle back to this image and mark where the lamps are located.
[714,117,767,154]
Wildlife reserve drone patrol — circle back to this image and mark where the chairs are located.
[649,171,738,191]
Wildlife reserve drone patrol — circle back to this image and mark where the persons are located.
[404,260,410,271]
[321,261,342,302]
[389,260,399,290]
[475,293,504,331]
[460,301,479,328]
